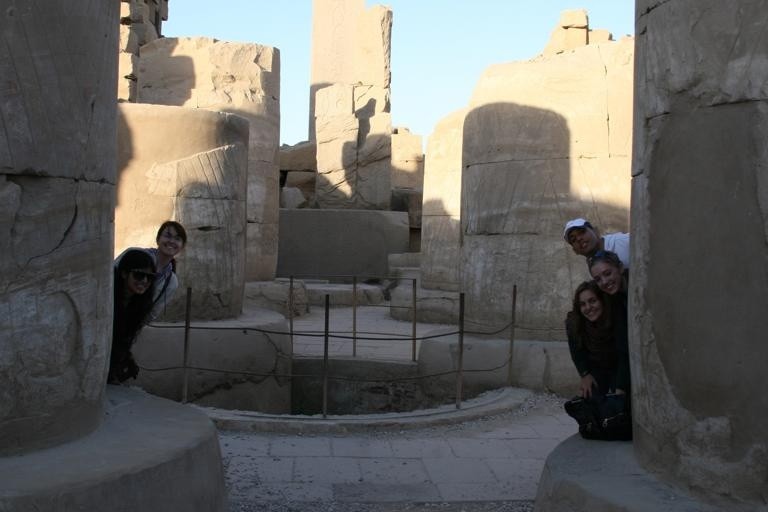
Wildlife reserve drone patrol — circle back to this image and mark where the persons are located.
[114,222,187,345]
[108,249,158,385]
[564,218,630,399]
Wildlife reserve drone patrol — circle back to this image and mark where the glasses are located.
[160,234,183,243]
[130,269,157,282]
[586,250,604,262]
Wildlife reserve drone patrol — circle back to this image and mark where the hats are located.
[563,218,586,243]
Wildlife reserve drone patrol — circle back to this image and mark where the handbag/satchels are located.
[565,392,632,424]
[116,352,140,383]
